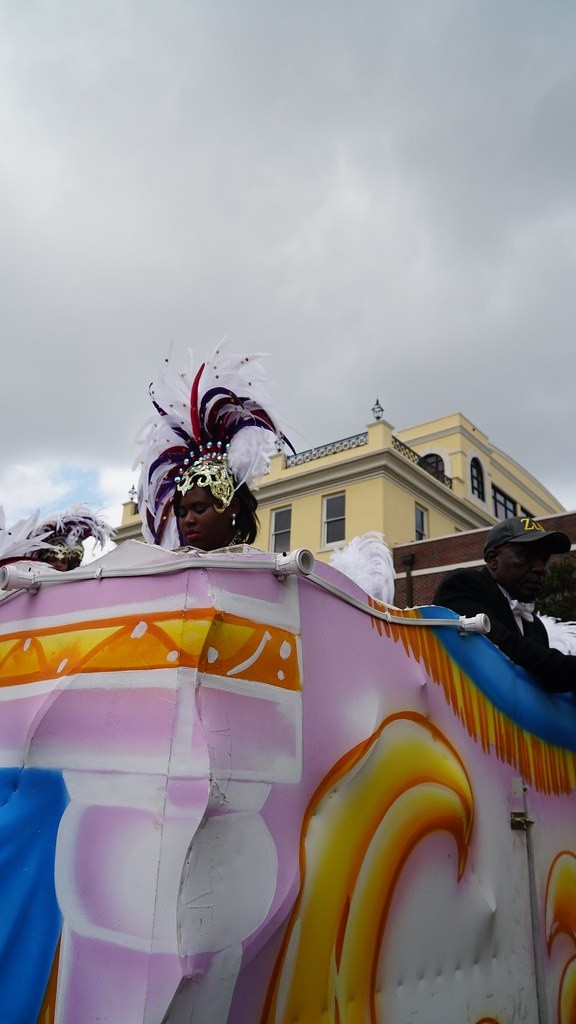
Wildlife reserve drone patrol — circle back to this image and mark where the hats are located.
[483,515,572,562]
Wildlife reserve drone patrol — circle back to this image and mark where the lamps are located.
[274,440,285,453]
[128,484,137,501]
[371,399,384,421]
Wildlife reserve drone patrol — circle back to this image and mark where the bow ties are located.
[510,598,536,623]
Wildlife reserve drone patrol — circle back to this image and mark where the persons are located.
[432,516,576,694]
[40,536,84,572]
[172,454,258,553]
